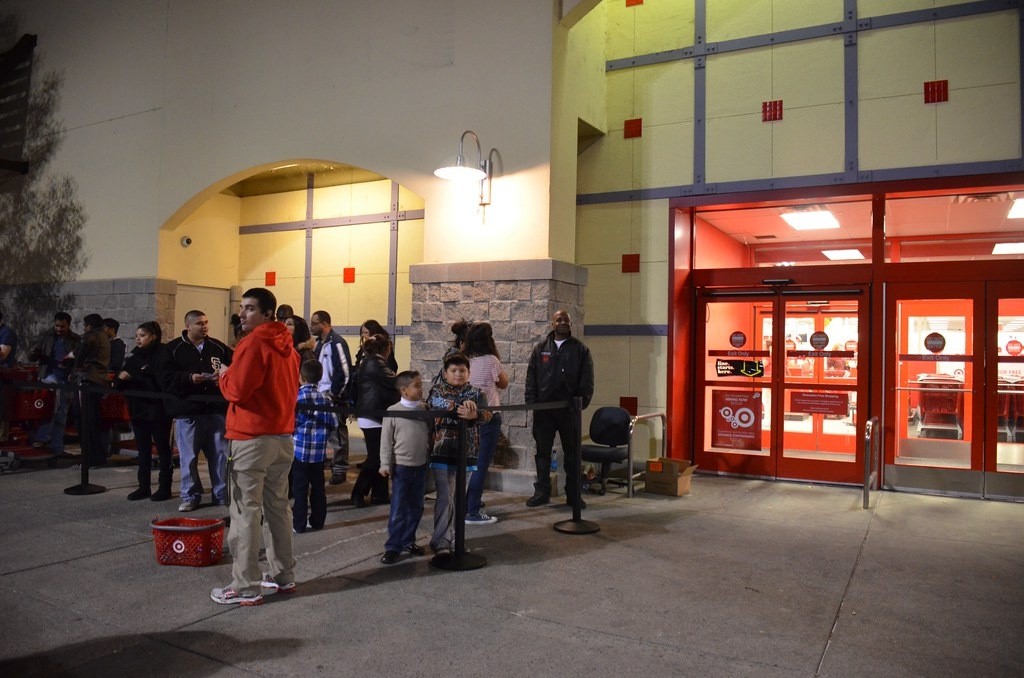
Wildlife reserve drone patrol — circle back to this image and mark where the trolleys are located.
[915,373,964,441]
[995,373,1024,442]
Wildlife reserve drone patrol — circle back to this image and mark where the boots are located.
[152,473,173,501]
[127,472,151,500]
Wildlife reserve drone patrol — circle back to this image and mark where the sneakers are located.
[261,572,296,593]
[567,498,586,509]
[465,511,497,525]
[526,494,549,506]
[211,585,265,606]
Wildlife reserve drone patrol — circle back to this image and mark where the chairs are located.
[581,406,635,497]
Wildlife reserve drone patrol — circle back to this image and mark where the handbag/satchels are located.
[341,371,356,404]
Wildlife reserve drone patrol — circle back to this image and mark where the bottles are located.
[550,450,558,472]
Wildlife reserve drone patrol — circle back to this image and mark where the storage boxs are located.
[644,456,700,497]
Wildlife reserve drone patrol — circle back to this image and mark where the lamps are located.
[434,129,494,208]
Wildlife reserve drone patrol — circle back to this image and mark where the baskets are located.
[151,514,224,567]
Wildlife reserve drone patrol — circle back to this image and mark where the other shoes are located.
[351,494,363,506]
[405,543,425,554]
[380,551,398,564]
[451,541,471,552]
[329,474,346,484]
[59,451,73,459]
[293,528,296,533]
[372,493,392,504]
[178,500,193,511]
[435,542,449,553]
[306,520,312,529]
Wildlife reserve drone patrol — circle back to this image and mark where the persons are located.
[209,288,397,607]
[0,314,22,365]
[429,352,493,556]
[68,313,127,466]
[445,318,510,526]
[827,342,847,371]
[525,309,594,509]
[111,321,174,501]
[26,312,81,448]
[166,309,229,512]
[801,357,814,376]
[378,371,430,565]
